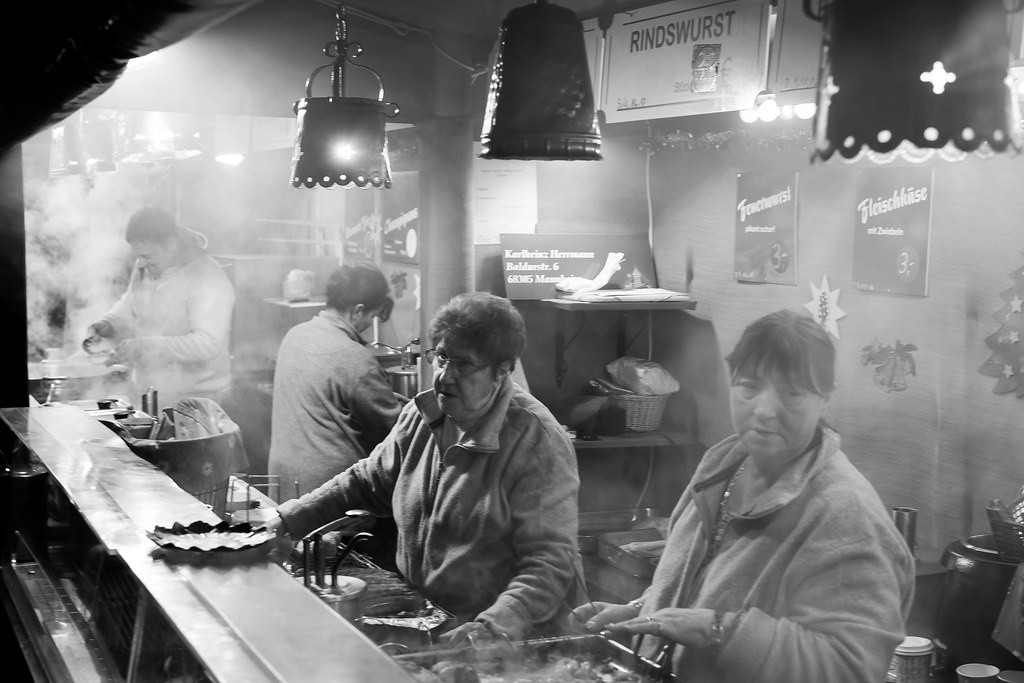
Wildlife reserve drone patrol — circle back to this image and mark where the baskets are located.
[593,384,672,431]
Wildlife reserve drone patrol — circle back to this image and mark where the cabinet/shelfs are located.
[529,281,696,449]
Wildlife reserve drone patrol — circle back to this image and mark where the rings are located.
[647,617,650,621]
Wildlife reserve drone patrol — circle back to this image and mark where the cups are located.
[997,670,1024,683]
[956,663,1000,683]
[894,636,934,683]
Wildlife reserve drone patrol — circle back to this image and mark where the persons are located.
[268,260,404,504]
[257,291,581,649]
[87,205,236,409]
[569,308,916,683]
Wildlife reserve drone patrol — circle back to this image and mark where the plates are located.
[146,519,278,553]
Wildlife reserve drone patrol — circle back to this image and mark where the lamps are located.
[798,0,1024,161]
[292,2,400,189]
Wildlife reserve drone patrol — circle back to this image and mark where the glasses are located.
[422,347,501,372]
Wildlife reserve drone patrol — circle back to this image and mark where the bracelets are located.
[711,611,725,652]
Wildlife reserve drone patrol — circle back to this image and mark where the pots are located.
[29,375,97,405]
